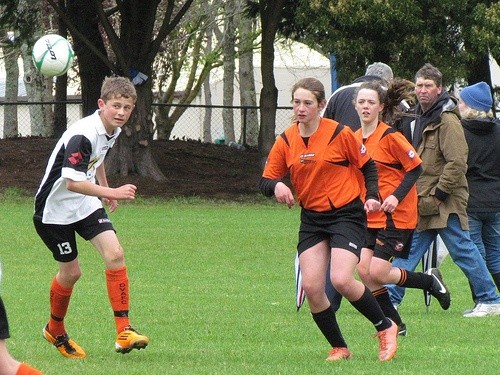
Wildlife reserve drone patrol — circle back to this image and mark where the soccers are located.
[31,34,76,77]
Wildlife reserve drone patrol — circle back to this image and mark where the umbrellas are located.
[422,233,449,313]
[294,250,305,311]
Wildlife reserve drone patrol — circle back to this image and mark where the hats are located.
[365,62,394,85]
[460,81,492,112]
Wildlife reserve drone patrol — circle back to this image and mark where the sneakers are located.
[325,348,352,361]
[462,303,500,318]
[373,317,398,362]
[42,323,86,359]
[424,267,450,310]
[397,323,406,336]
[115,327,150,354]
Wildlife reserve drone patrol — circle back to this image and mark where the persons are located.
[33,76,150,360]
[322,63,500,318]
[350,80,452,337]
[259,77,399,363]
